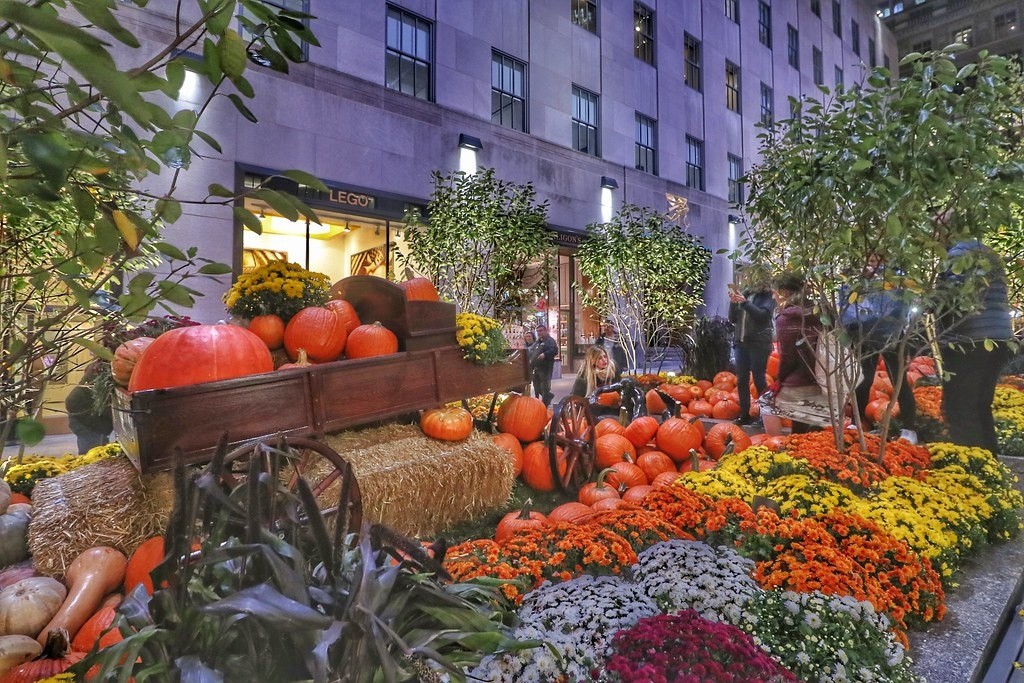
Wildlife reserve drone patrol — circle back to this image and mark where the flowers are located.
[455,310,509,368]
[222,258,331,320]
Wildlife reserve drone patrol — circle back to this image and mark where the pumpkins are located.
[397,268,439,301]
[598,352,793,429]
[842,354,936,421]
[483,382,786,543]
[388,540,436,573]
[0,479,202,683]
[420,406,473,441]
[111,300,398,392]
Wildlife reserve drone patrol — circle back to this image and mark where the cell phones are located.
[728,283,738,295]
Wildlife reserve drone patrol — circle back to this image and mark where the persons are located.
[524,325,558,409]
[66,358,113,459]
[568,345,622,398]
[838,252,919,445]
[595,320,628,376]
[758,271,835,437]
[922,208,1014,460]
[728,286,774,429]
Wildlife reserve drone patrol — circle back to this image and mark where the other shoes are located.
[733,411,751,425]
[751,418,764,426]
[542,392,554,407]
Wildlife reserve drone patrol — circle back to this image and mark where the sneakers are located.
[847,420,865,430]
[900,429,918,445]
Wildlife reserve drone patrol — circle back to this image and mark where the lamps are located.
[343,221,350,232]
[728,215,741,225]
[600,176,619,190]
[375,224,380,236]
[458,133,483,152]
[395,230,400,237]
[258,209,266,220]
